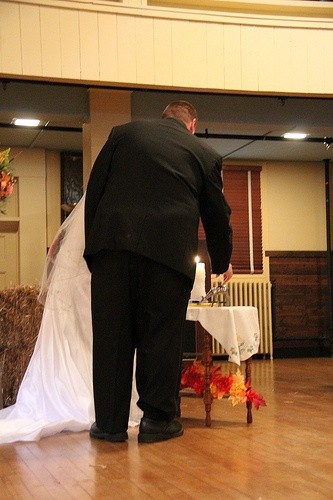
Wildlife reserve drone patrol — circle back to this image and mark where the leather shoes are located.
[138,419,184,443]
[90,422,128,442]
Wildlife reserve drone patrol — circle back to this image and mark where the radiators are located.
[211,274,273,360]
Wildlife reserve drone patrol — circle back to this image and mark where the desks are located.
[179,305,260,428]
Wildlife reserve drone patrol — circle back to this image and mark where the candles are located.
[188,255,207,302]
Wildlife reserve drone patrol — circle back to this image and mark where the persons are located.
[40,188,94,425]
[83,98,236,442]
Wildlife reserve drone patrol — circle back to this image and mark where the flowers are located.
[0,147,17,200]
